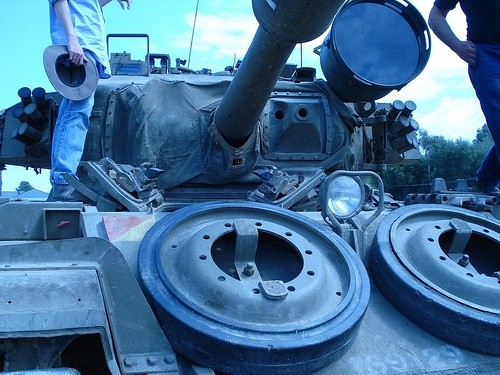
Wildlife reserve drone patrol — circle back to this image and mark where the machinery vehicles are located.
[0,0,500,375]
